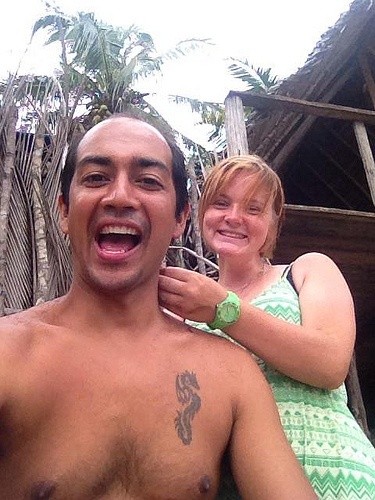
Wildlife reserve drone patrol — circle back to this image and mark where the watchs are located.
[206,291,239,330]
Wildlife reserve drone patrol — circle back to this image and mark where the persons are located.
[158,155,375,500]
[0,111,319,500]
